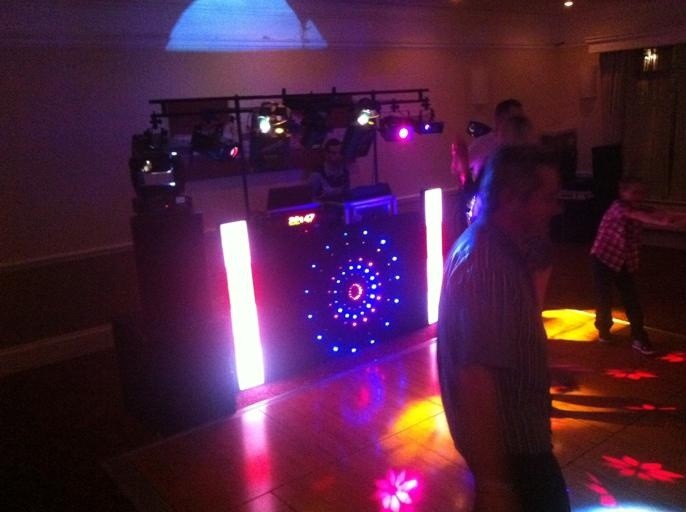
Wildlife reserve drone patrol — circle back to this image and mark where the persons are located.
[436,100,571,512]
[589,178,678,355]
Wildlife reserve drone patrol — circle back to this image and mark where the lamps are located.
[136,96,441,180]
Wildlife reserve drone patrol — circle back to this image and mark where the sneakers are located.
[632,340,656,355]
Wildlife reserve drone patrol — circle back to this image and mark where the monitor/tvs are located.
[267,184,312,210]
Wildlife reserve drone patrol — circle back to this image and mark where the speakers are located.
[112,312,236,431]
[542,132,576,183]
[133,225,212,359]
[592,144,621,233]
